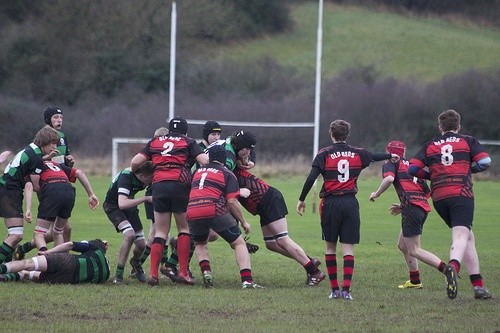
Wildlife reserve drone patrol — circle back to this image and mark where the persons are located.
[102,121,260,286]
[369,140,461,289]
[186,145,264,289]
[408,109,493,300]
[297,120,400,301]
[1,239,111,284]
[24,156,99,255]
[138,117,211,286]
[233,167,326,287]
[0,127,60,265]
[14,106,76,261]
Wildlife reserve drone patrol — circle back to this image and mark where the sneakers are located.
[342,290,354,302]
[327,289,340,301]
[240,235,259,254]
[128,267,138,278]
[129,258,148,283]
[200,271,214,288]
[308,270,325,287]
[12,244,25,262]
[112,277,123,286]
[175,268,198,285]
[473,285,492,300]
[305,255,321,273]
[159,262,181,284]
[147,276,160,286]
[397,279,424,290]
[442,263,459,299]
[241,281,267,291]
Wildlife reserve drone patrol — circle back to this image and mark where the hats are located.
[44,105,64,127]
[202,119,223,142]
[385,140,407,160]
[231,131,257,156]
[168,116,189,135]
[208,145,228,166]
